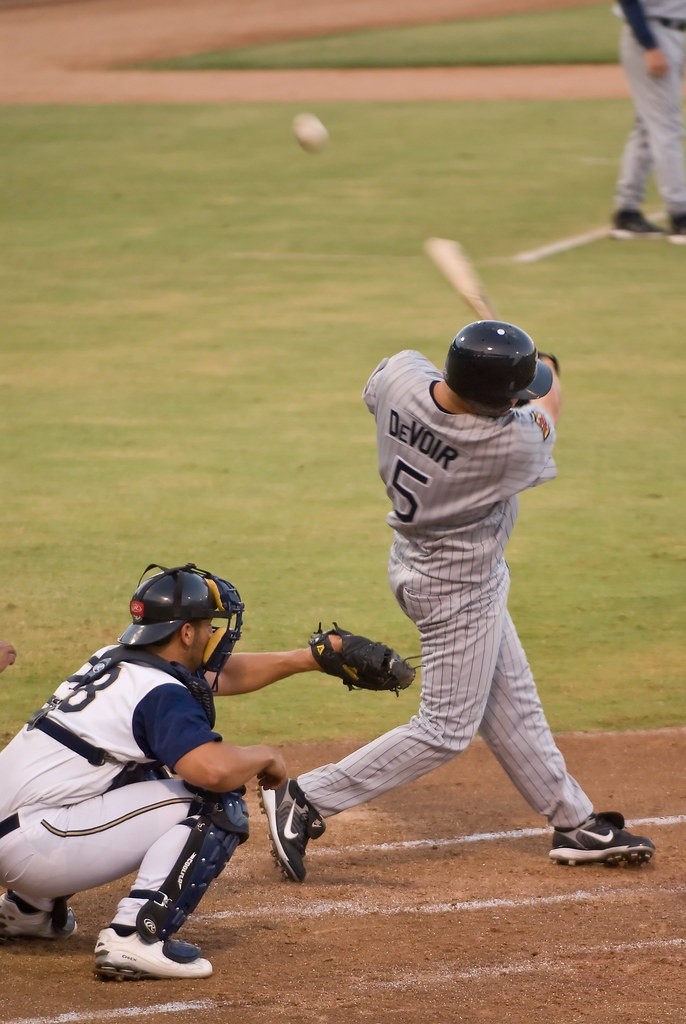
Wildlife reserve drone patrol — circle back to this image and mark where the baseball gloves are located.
[306,621,415,697]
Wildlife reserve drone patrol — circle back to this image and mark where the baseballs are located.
[294,115,326,152]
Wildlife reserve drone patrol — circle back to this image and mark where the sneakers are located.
[255,778,326,883]
[0,890,78,941]
[93,929,213,982]
[666,213,686,245]
[609,209,666,240]
[548,811,656,868]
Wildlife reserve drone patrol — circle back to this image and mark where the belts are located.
[0,813,20,839]
[659,17,686,32]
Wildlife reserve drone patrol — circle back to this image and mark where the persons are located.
[256,320,657,883]
[609,0,686,245]
[0,562,433,980]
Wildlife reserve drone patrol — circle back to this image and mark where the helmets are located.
[117,568,217,646]
[442,321,553,412]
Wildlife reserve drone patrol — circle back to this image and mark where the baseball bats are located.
[422,236,494,320]
